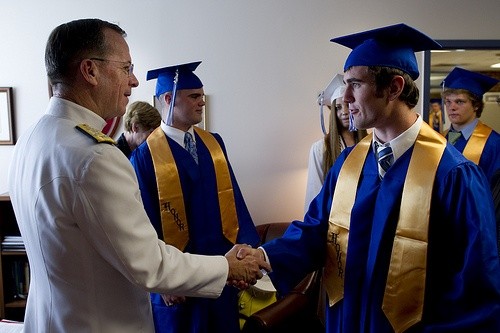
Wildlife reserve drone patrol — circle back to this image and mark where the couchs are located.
[240,221,320,333]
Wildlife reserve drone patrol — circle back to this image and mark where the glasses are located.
[90,57,134,77]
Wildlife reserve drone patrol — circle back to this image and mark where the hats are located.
[147,62,203,126]
[316,73,345,136]
[440,67,500,124]
[330,24,442,132]
[429,98,441,105]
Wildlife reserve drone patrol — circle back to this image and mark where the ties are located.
[376,143,394,182]
[184,132,198,165]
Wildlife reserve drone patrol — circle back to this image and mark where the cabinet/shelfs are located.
[0,192,30,321]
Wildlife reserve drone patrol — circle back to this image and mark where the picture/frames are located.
[0,87,16,145]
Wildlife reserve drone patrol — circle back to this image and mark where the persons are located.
[115,101,162,161]
[303,73,369,220]
[440,67,500,225]
[130,61,261,333]
[228,23,500,333]
[10,18,272,333]
[429,98,444,134]
